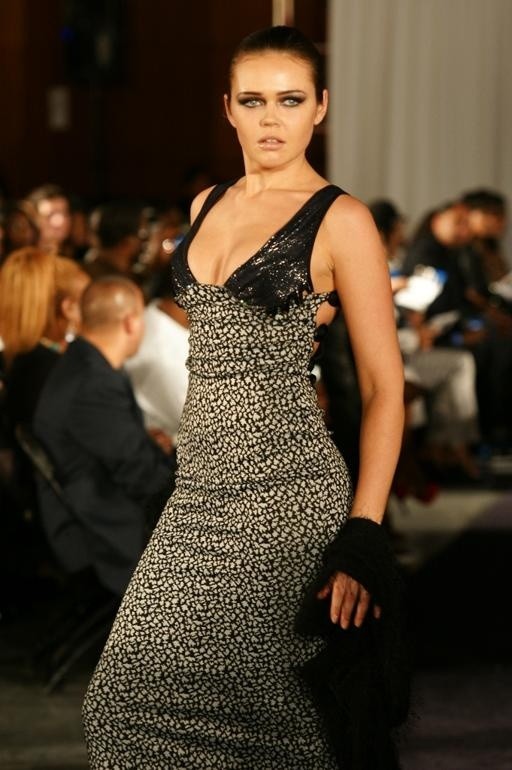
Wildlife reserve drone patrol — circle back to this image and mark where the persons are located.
[0,181,512,604]
[80,24,408,769]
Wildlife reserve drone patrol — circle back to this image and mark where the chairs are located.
[11,414,127,701]
